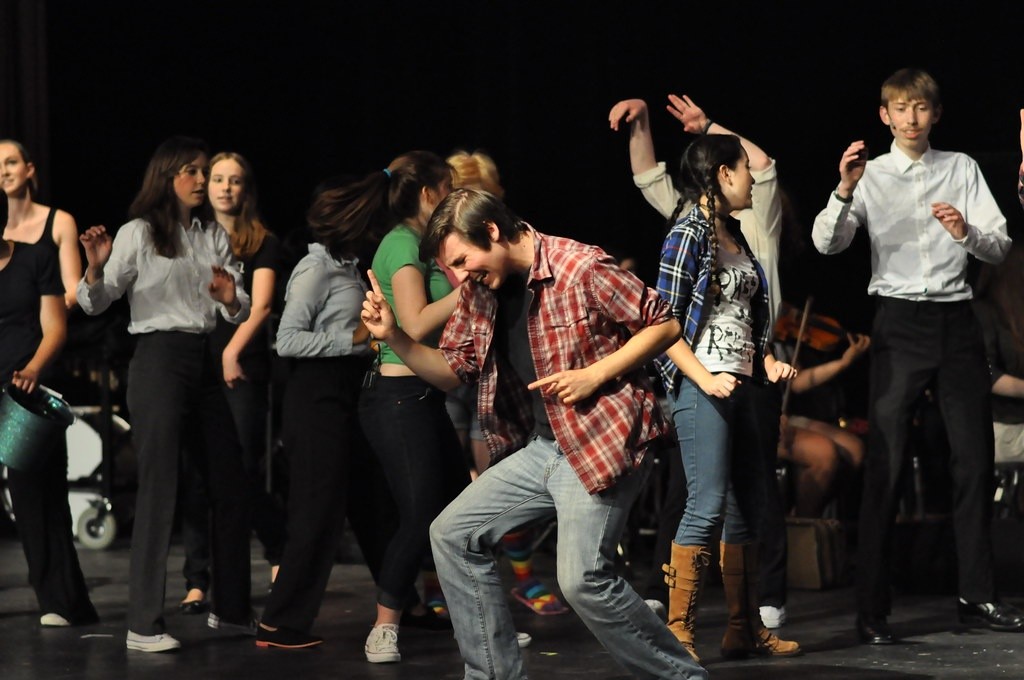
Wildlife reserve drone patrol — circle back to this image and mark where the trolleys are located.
[0,350,137,551]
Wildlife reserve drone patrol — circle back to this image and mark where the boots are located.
[717,540,804,656]
[661,539,713,663]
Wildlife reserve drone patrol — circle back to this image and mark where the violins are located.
[773,304,858,352]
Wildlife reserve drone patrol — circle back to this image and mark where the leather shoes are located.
[254,623,325,648]
[856,613,896,645]
[957,593,1024,631]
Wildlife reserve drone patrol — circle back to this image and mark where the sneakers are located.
[364,623,402,665]
[41,612,71,627]
[126,626,183,652]
[517,632,533,649]
[759,605,787,629]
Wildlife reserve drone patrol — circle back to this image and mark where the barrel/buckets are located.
[0,378,75,471]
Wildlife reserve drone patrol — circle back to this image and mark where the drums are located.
[2,407,131,537]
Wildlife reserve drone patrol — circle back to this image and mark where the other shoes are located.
[207,610,259,637]
[180,586,207,614]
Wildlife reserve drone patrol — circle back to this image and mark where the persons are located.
[609,95,788,629]
[971,246,1024,462]
[0,189,101,627]
[424,150,569,615]
[764,332,870,517]
[359,189,710,680]
[655,134,801,665]
[256,187,373,648]
[0,140,81,309]
[811,68,1024,644]
[310,155,531,662]
[76,142,260,652]
[180,152,284,613]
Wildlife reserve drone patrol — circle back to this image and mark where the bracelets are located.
[834,189,853,204]
[702,119,713,134]
